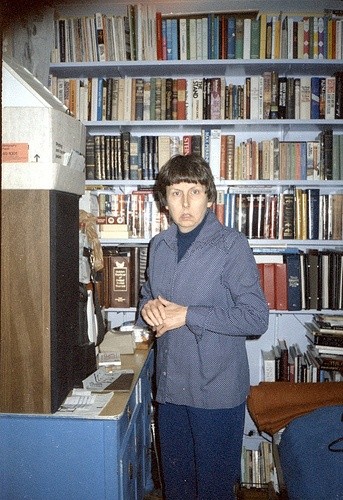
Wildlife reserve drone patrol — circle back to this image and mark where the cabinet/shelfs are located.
[42,6,343,489]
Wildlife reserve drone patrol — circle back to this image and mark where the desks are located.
[0,332,159,500]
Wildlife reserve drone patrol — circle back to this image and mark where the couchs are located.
[278,403,343,500]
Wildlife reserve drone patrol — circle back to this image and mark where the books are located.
[258,244,342,308]
[136,153,270,500]
[240,434,286,492]
[50,0,342,65]
[210,187,343,241]
[47,74,343,120]
[97,185,173,241]
[262,312,342,382]
[92,247,147,310]
[83,128,343,182]
